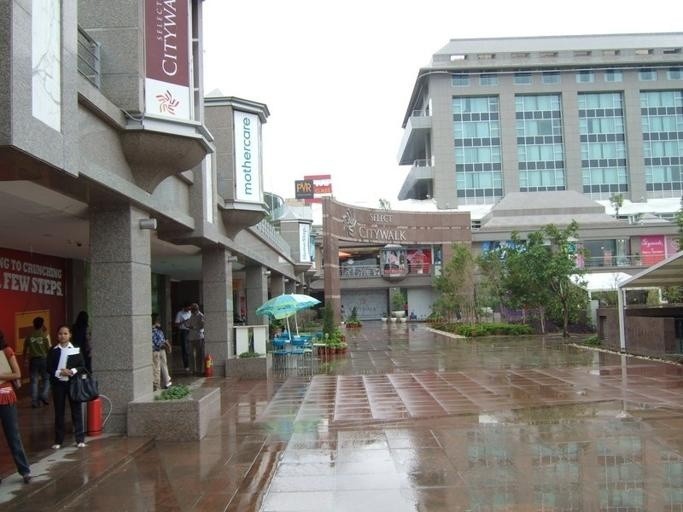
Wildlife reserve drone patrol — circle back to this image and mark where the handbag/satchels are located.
[70,373,99,403]
[12,379,23,390]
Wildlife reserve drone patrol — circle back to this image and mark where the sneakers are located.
[164,382,171,389]
[51,444,61,449]
[31,400,41,408]
[23,472,33,482]
[77,442,86,448]
[38,394,50,405]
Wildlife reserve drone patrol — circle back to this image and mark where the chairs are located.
[270,333,327,361]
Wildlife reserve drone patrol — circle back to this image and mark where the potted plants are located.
[318,333,348,357]
[382,293,407,323]
[345,320,361,328]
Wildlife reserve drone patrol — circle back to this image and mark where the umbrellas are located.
[290,292,321,336]
[254,292,315,339]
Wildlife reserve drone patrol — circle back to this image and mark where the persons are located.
[174,302,192,372]
[69,309,91,371]
[0,328,32,483]
[408,249,427,274]
[46,326,89,449]
[22,316,54,407]
[183,303,206,377]
[387,251,398,264]
[340,304,345,320]
[150,312,172,389]
[403,301,407,316]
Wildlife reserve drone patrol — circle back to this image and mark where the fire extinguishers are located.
[88,394,112,435]
[205,353,213,377]
